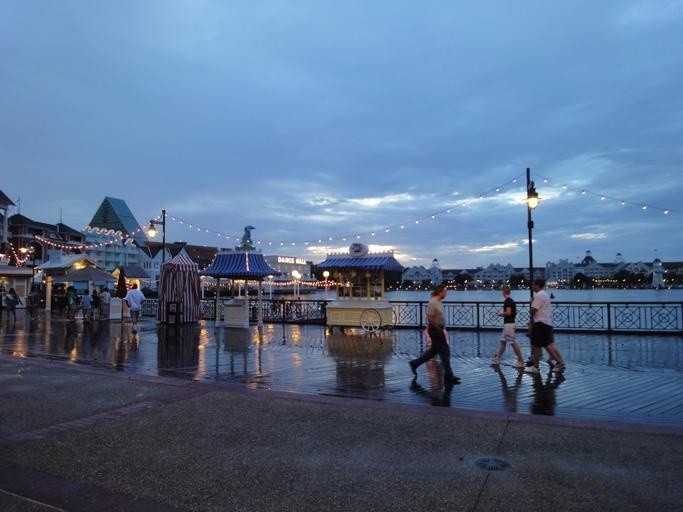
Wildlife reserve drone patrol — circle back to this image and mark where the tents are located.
[157,324,199,377]
[158,253,200,324]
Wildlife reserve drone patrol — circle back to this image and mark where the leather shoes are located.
[409,361,417,375]
[444,375,460,380]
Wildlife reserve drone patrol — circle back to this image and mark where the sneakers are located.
[489,356,566,372]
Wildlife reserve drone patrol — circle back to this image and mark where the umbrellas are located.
[116,268,128,320]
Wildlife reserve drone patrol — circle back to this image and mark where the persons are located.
[410,361,565,416]
[125,284,146,333]
[127,329,140,367]
[4,286,111,323]
[408,279,566,381]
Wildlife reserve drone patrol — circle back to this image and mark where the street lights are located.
[322,270,330,294]
[526,167,542,338]
[17,232,36,286]
[146,208,167,264]
[268,274,273,300]
[291,270,302,299]
